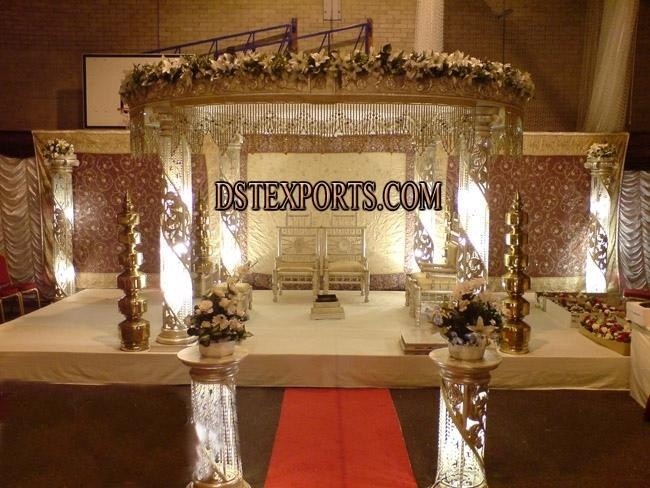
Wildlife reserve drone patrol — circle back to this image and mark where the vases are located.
[449,343,488,359]
[196,337,236,359]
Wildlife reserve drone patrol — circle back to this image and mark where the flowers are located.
[586,143,619,161]
[41,139,75,158]
[183,282,252,343]
[425,276,506,345]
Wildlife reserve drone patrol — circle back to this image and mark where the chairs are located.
[0,252,42,323]
[405,241,462,328]
[271,210,370,303]
